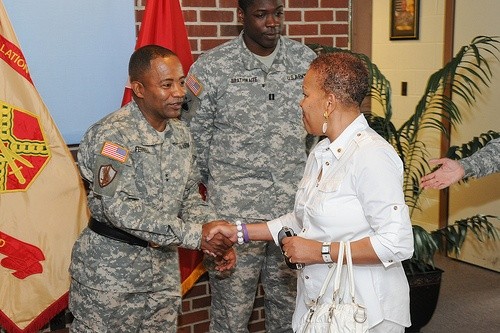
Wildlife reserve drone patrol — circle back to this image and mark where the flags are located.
[122,0,207,298]
[0,1,90,333]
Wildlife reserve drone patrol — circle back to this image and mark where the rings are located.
[288,258,292,263]
[284,251,288,257]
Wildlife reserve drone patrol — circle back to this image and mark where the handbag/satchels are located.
[297,241,371,333]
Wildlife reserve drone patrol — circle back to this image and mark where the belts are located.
[88,215,159,250]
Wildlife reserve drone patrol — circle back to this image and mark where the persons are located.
[421,137,500,191]
[208,51,415,333]
[183,0,319,333]
[68,46,236,333]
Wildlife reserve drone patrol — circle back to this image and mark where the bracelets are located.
[322,242,334,264]
[242,223,249,243]
[236,220,244,245]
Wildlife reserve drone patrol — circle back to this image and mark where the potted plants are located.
[306,35,500,333]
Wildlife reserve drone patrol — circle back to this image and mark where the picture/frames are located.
[389,0,419,40]
